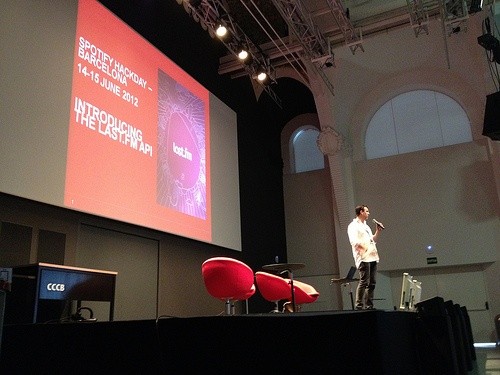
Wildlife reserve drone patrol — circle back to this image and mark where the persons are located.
[347,205,384,311]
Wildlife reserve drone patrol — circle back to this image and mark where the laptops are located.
[341,266,356,280]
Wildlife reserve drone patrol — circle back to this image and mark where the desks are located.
[3,261,118,323]
[262,263,306,278]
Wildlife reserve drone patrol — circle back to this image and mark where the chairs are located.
[201,257,254,315]
[292,280,321,312]
[220,283,256,315]
[255,271,295,314]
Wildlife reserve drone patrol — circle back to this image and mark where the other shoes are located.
[364,307,376,311]
[356,306,363,312]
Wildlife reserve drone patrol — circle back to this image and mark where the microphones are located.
[372,218,385,229]
